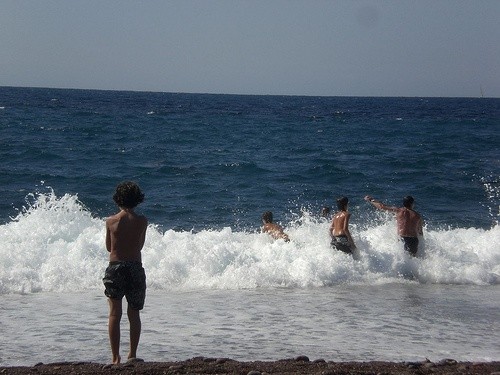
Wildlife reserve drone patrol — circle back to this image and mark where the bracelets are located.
[370,198,375,204]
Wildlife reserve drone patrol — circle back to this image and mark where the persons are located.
[318,206,331,223]
[329,196,357,260]
[102,180,147,365]
[364,195,424,257]
[260,211,291,243]
